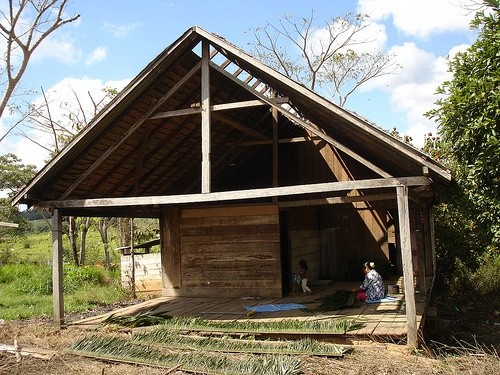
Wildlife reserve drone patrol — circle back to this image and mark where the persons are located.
[295,259,313,296]
[344,288,358,306]
[354,261,386,307]
